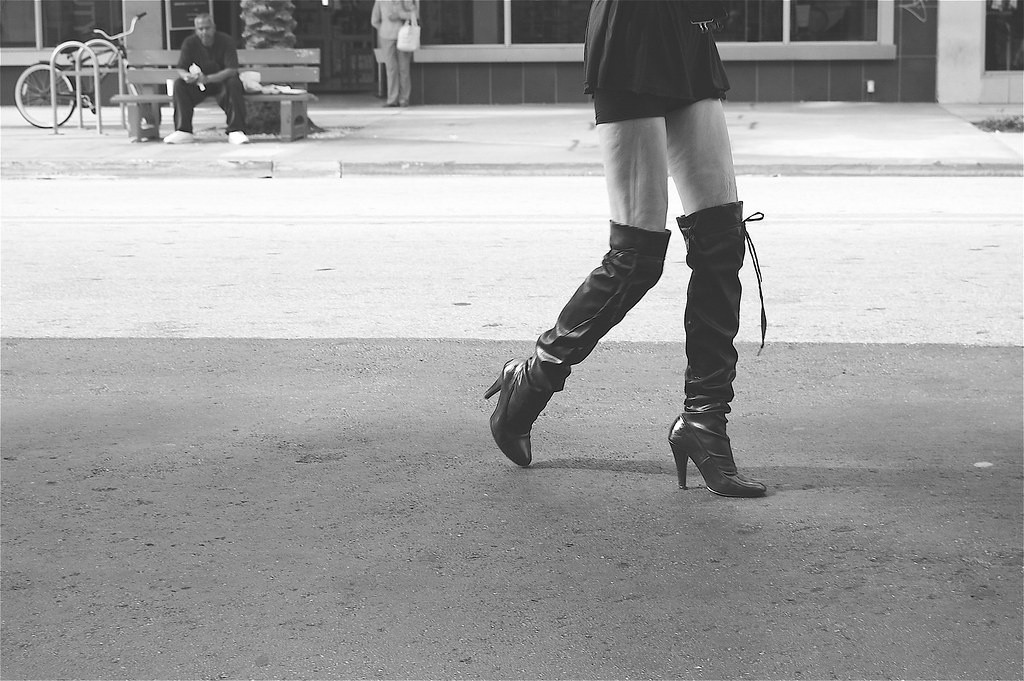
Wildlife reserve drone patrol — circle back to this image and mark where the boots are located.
[483,219,672,467]
[667,199,768,498]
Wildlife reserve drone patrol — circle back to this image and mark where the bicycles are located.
[14,12,147,129]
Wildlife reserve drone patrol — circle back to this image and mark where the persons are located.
[371,0,419,108]
[163,14,250,144]
[486,1,768,497]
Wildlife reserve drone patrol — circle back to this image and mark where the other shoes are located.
[228,131,251,145]
[164,130,196,145]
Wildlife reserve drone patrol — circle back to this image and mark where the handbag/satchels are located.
[397,9,422,53]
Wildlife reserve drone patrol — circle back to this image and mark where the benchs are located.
[108,47,322,144]
